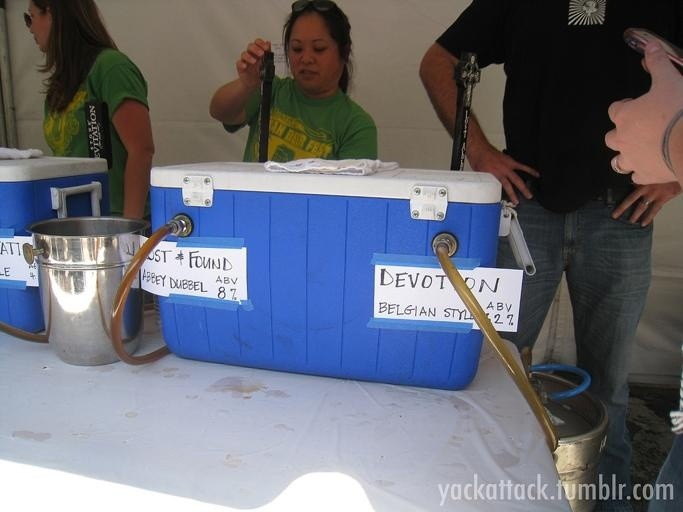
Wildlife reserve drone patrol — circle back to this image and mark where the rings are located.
[638,197,653,209]
[609,155,631,175]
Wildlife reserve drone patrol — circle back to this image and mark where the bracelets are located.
[660,107,682,186]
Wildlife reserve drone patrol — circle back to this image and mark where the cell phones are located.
[623,28,683,71]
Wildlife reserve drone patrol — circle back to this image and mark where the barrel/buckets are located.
[535,371,610,511]
[24,216,152,367]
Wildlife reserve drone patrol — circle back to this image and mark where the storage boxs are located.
[150,160,503,391]
[0,157,108,333]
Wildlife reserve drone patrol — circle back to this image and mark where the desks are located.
[0,309,573,512]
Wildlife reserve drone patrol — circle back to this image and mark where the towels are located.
[264,158,401,176]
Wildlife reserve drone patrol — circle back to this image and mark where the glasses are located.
[24,7,50,27]
[292,1,338,14]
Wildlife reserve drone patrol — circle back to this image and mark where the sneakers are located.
[599,497,632,511]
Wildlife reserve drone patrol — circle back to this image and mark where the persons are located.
[207,1,379,162]
[602,22,682,512]
[20,0,156,222]
[416,0,683,512]
